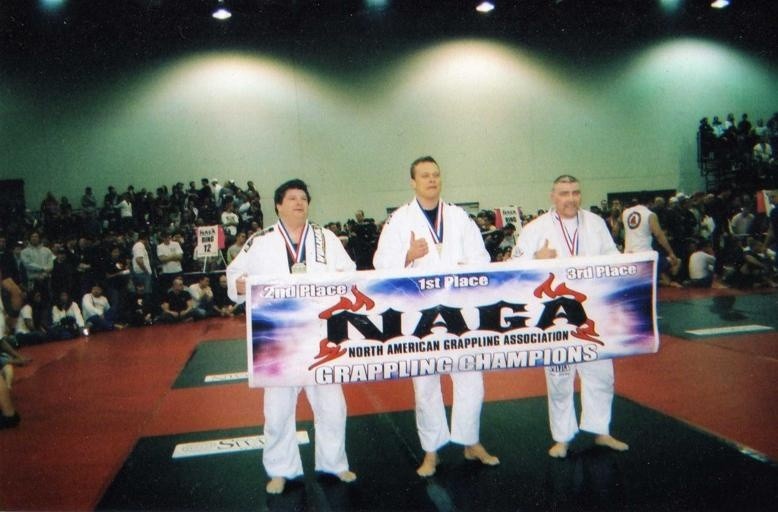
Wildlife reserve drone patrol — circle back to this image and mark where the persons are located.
[371,155,502,481]
[1,177,266,345]
[697,110,777,301]
[220,178,357,496]
[0,364,19,393]
[316,191,697,303]
[505,174,632,463]
[0,338,33,368]
[0,374,22,431]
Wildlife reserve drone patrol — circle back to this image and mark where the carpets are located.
[171,338,247,387]
[91,388,778,512]
[657,291,778,340]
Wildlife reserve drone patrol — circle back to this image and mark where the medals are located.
[290,263,306,275]
[435,243,443,255]
